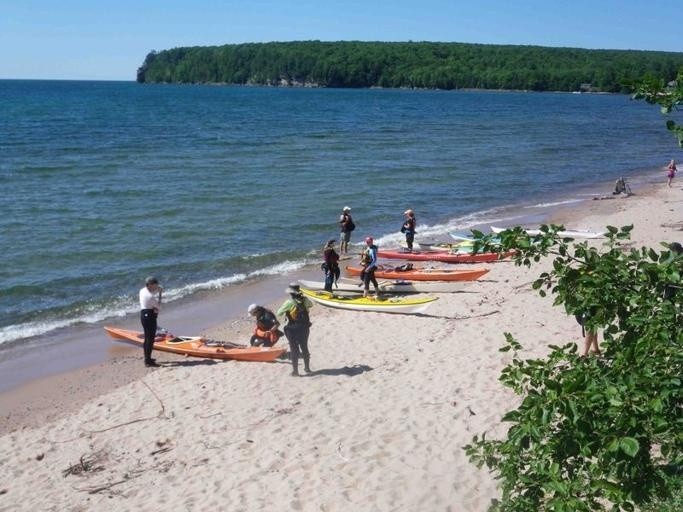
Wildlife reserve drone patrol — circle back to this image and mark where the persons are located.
[324,239,334,293]
[404,210,416,251]
[247,304,284,347]
[582,323,598,353]
[363,237,379,298]
[276,284,313,376]
[340,206,355,253]
[139,276,161,366]
[358,251,369,287]
[616,177,625,192]
[331,249,339,288]
[667,160,677,186]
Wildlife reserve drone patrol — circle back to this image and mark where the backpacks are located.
[289,298,309,324]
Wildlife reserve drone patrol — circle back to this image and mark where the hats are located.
[343,206,351,211]
[248,303,260,317]
[404,209,413,215]
[286,283,303,296]
